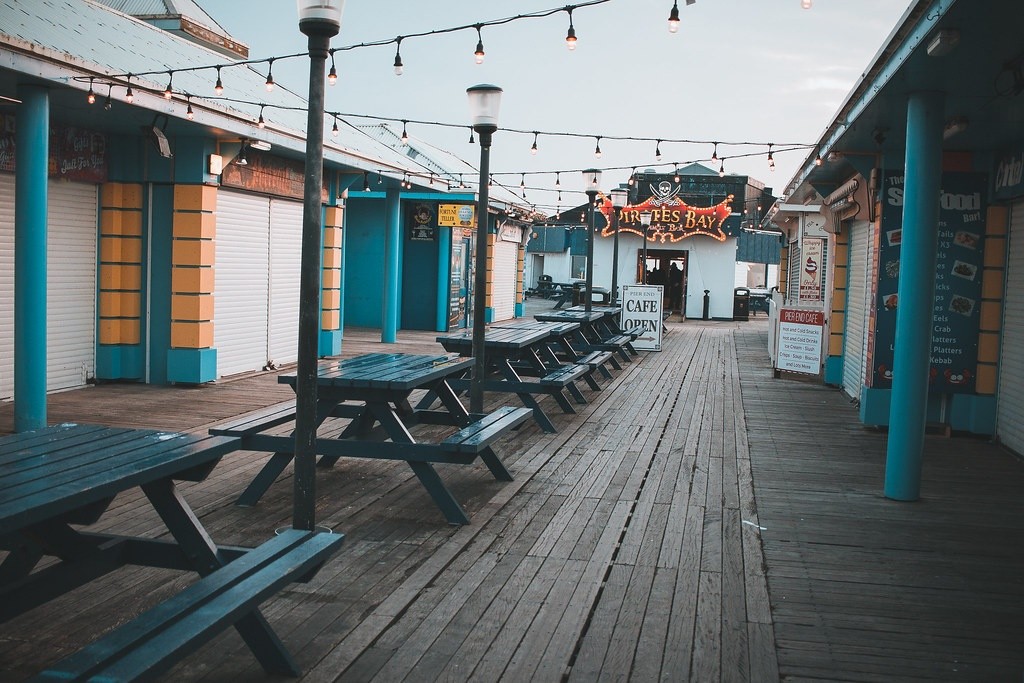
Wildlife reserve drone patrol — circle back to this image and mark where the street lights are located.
[468,84,504,413]
[611,186,630,308]
[294,0,349,533]
[640,211,652,284]
[582,167,603,312]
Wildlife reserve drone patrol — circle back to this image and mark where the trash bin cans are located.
[579,287,611,306]
[572,280,586,307]
[734,287,750,321]
[539,275,552,291]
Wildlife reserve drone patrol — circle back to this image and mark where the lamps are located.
[340,188,349,199]
[563,225,575,231]
[235,134,250,166]
[828,148,846,164]
[361,172,371,192]
[532,232,537,238]
[207,154,223,176]
[140,113,173,157]
[250,138,272,154]
[927,29,959,56]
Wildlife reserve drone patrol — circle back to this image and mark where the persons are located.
[645,262,684,310]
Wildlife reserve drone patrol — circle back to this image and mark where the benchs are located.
[529,286,592,299]
[0,310,673,683]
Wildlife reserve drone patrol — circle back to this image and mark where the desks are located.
[490,322,616,391]
[565,305,638,356]
[234,352,514,528]
[0,423,302,683]
[534,312,633,372]
[435,328,576,435]
[529,280,577,309]
